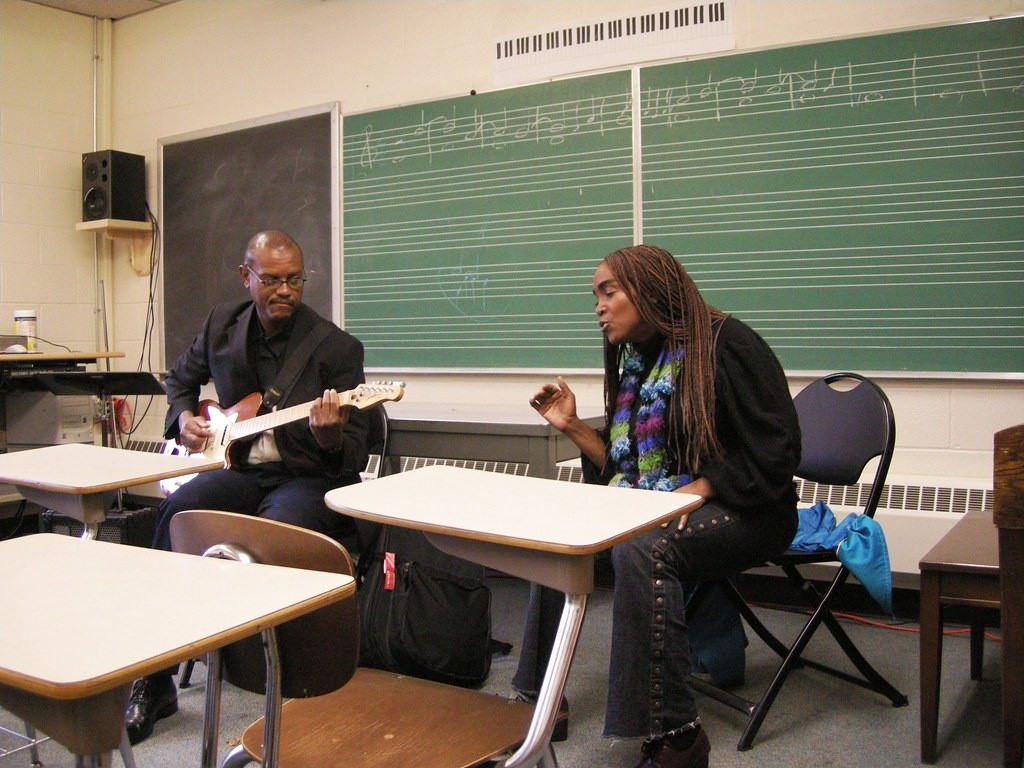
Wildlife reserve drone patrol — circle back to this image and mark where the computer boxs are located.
[5,384,94,444]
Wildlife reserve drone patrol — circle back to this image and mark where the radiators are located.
[96,412,170,499]
[547,447,993,575]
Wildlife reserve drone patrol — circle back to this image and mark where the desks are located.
[918,510,997,761]
[324,464,705,768]
[0,443,225,540]
[0,534,354,768]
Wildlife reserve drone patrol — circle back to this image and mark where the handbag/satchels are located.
[354,553,513,688]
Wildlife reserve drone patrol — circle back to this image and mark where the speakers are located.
[82,150,146,223]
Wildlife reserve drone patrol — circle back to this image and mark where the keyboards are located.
[7,367,87,376]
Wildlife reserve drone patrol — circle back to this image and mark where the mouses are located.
[5,344,27,354]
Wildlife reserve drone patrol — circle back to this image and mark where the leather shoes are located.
[488,692,568,761]
[124,678,179,746]
[634,724,712,768]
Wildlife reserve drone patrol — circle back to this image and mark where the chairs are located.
[166,370,910,768]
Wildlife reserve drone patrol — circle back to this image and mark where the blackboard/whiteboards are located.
[155,100,341,375]
[337,14,1024,380]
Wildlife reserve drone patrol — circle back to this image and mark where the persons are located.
[514,244,802,768]
[124,230,369,746]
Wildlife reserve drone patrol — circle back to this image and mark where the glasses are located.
[245,264,307,290]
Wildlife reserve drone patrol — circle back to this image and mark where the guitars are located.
[159,381,406,495]
